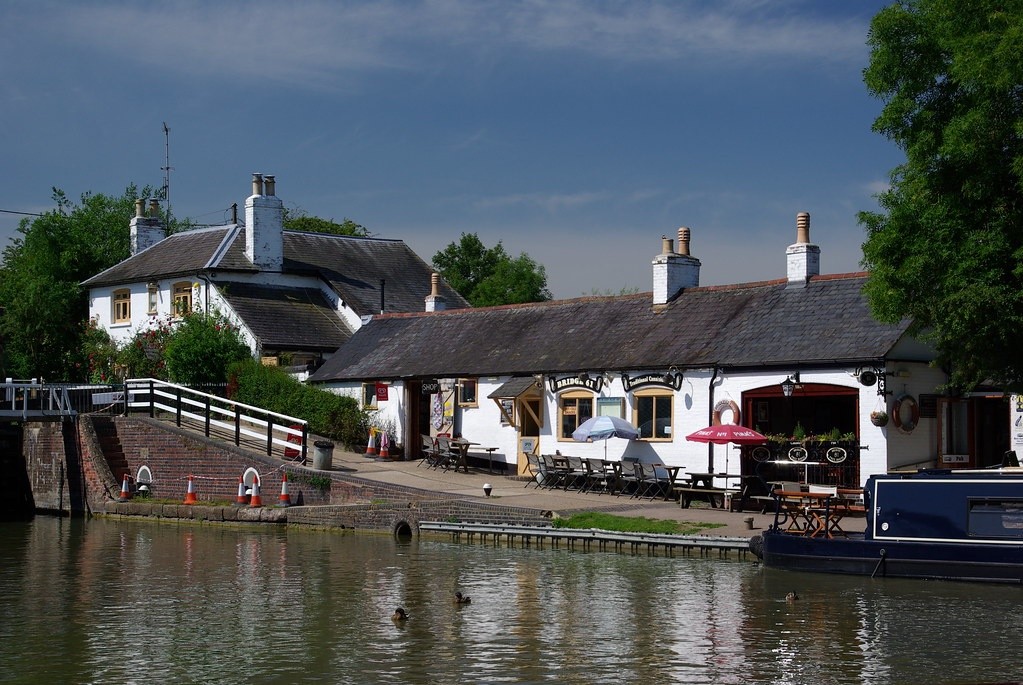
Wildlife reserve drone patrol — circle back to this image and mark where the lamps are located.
[487,376,500,382]
[90,316,98,327]
[604,370,623,383]
[532,373,544,388]
[662,365,684,386]
[577,370,589,382]
[780,371,801,398]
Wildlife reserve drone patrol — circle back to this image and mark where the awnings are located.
[487,375,544,429]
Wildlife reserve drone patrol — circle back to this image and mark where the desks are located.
[776,489,833,533]
[441,440,480,471]
[656,466,685,501]
[682,472,748,507]
[804,503,845,539]
[763,481,809,513]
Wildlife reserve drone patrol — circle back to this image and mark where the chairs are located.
[434,438,460,471]
[523,453,670,502]
[417,434,439,469]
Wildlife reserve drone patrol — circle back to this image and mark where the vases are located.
[871,416,888,426]
[176,312,181,317]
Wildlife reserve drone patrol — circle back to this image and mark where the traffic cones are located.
[275,472,294,507]
[245,475,267,508]
[182,474,197,504]
[229,475,249,506]
[118,473,132,502]
[362,428,379,459]
[374,431,393,463]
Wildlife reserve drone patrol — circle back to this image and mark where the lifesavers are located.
[893,394,920,434]
[714,400,740,427]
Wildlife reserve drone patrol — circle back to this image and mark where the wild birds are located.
[454,592,471,603]
[391,607,409,620]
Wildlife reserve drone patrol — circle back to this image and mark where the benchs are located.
[749,495,822,500]
[671,483,746,494]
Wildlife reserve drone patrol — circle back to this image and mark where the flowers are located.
[172,300,184,312]
[873,412,889,418]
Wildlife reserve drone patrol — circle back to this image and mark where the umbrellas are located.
[571,415,641,474]
[686,423,768,510]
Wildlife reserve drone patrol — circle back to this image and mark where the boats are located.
[750,467,1023,584]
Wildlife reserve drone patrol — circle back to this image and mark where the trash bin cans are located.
[314,441,334,470]
